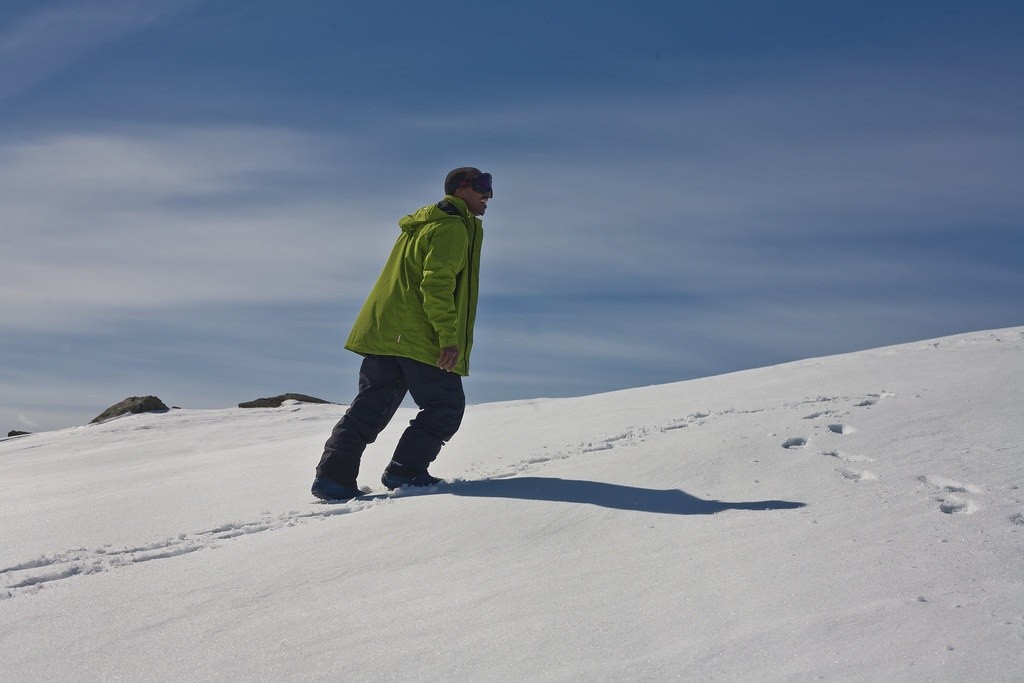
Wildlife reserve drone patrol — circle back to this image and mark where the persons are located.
[311,167,493,501]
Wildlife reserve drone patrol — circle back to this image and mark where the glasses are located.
[449,173,493,199]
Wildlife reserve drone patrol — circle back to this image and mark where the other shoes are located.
[380,462,440,489]
[311,476,366,501]
